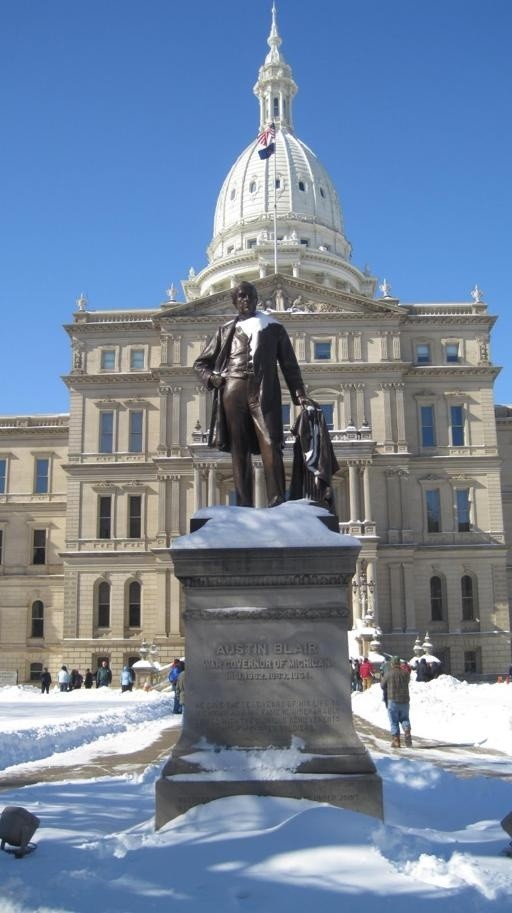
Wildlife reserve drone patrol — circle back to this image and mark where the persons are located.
[68,668,76,691]
[168,657,182,714]
[95,660,112,688]
[39,665,51,693]
[256,282,315,313]
[56,665,70,691]
[192,278,321,509]
[119,665,134,692]
[83,667,92,688]
[72,669,82,688]
[381,656,412,748]
[349,656,444,692]
[175,670,185,706]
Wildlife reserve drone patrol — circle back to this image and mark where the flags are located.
[257,143,276,159]
[256,122,276,146]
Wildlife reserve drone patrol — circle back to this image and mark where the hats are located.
[389,655,401,665]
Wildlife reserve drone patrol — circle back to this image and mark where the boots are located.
[404,729,412,747]
[390,735,402,748]
